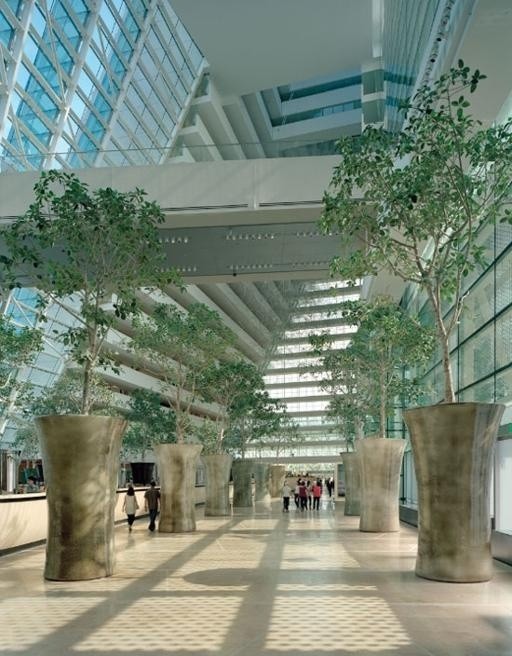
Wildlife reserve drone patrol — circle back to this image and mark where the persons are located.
[120,486,139,535]
[143,480,162,533]
[280,475,334,514]
[22,475,39,493]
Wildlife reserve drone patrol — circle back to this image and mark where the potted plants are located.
[306,293,441,533]
[0,169,185,590]
[175,356,305,517]
[118,297,237,536]
[300,58,512,582]
